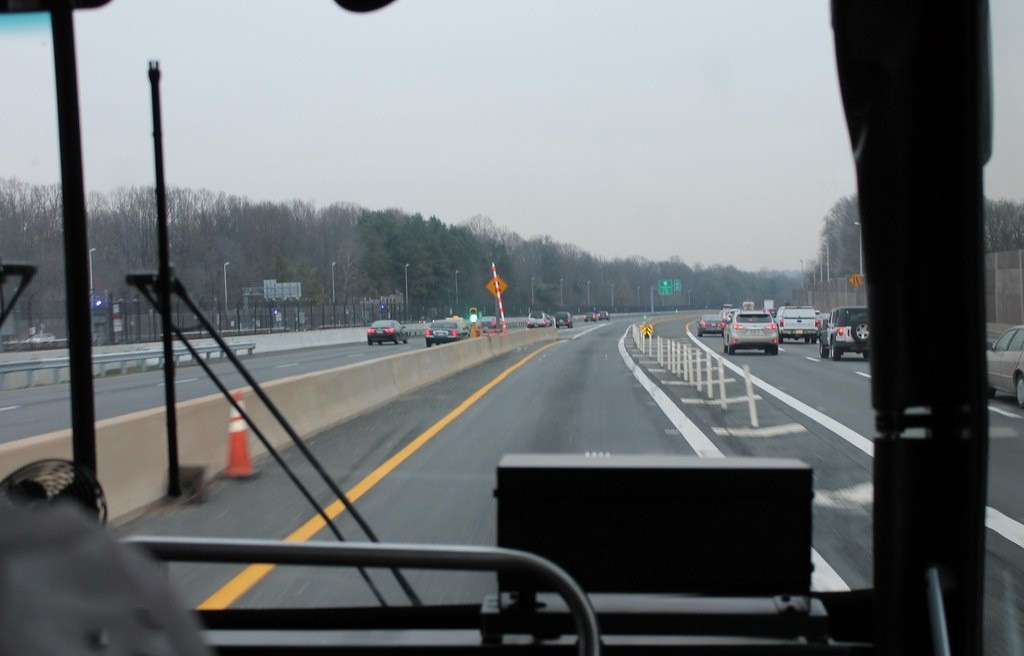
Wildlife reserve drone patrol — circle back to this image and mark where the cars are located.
[986,325,1024,409]
[763,308,778,318]
[424,316,499,347]
[367,319,408,345]
[697,313,723,337]
[584,310,609,322]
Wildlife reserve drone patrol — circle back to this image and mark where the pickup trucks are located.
[776,305,820,344]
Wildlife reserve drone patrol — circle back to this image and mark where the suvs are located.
[527,310,573,329]
[723,309,779,356]
[818,307,870,361]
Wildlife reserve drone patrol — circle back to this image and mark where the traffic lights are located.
[469,307,477,323]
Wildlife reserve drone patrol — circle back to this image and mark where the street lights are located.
[332,261,336,302]
[800,243,829,288]
[405,263,410,315]
[224,262,229,310]
[89,248,96,306]
[854,221,862,274]
[455,270,640,308]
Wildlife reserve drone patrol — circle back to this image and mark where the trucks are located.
[741,300,774,309]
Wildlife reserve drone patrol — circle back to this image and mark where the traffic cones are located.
[214,391,261,481]
